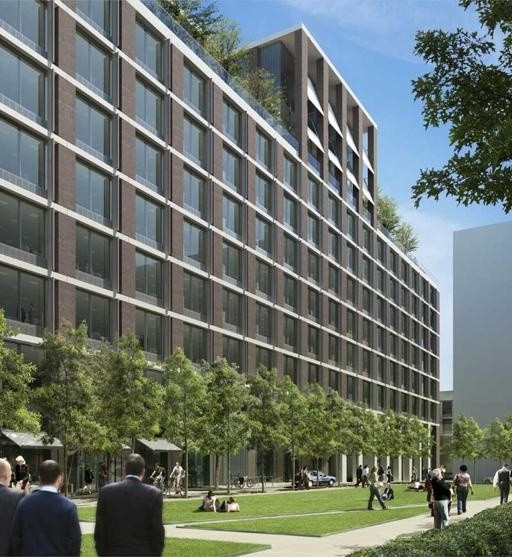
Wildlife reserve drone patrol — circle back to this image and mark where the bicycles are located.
[150,476,169,495]
[233,471,254,487]
[170,477,186,497]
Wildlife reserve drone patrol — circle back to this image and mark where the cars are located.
[295,469,337,486]
[482,475,512,484]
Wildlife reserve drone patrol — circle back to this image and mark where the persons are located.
[298,465,309,488]
[354,463,394,510]
[150,463,167,489]
[220,496,240,512]
[452,465,474,515]
[170,462,183,493]
[202,491,219,512]
[94,453,165,557]
[15,455,29,489]
[10,460,81,557]
[0,458,25,557]
[424,468,453,530]
[493,464,512,505]
[81,463,94,494]
